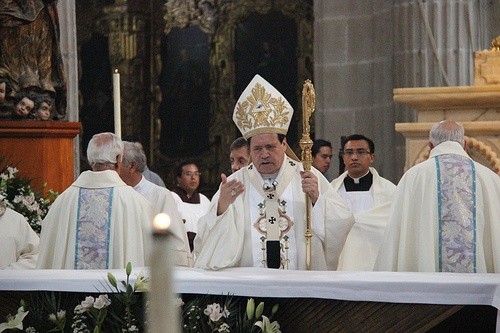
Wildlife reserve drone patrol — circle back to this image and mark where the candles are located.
[113,68,121,141]
[148,213,178,333]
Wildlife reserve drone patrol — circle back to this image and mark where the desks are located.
[1,269,499,333]
[0,121,82,206]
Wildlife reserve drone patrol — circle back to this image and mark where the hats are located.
[232,74,294,141]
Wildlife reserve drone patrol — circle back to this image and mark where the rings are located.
[231,189,238,198]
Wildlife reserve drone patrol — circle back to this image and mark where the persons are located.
[13,90,41,118]
[193,118,355,271]
[142,164,167,189]
[169,160,210,254]
[0,198,40,270]
[372,120,500,273]
[0,77,12,104]
[310,138,333,174]
[116,141,192,267]
[34,96,55,121]
[229,137,250,174]
[35,132,156,270]
[330,134,397,271]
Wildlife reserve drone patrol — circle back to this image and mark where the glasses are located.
[343,149,371,157]
[181,171,201,176]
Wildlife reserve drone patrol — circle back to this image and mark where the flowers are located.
[1,152,59,236]
[1,262,283,333]
